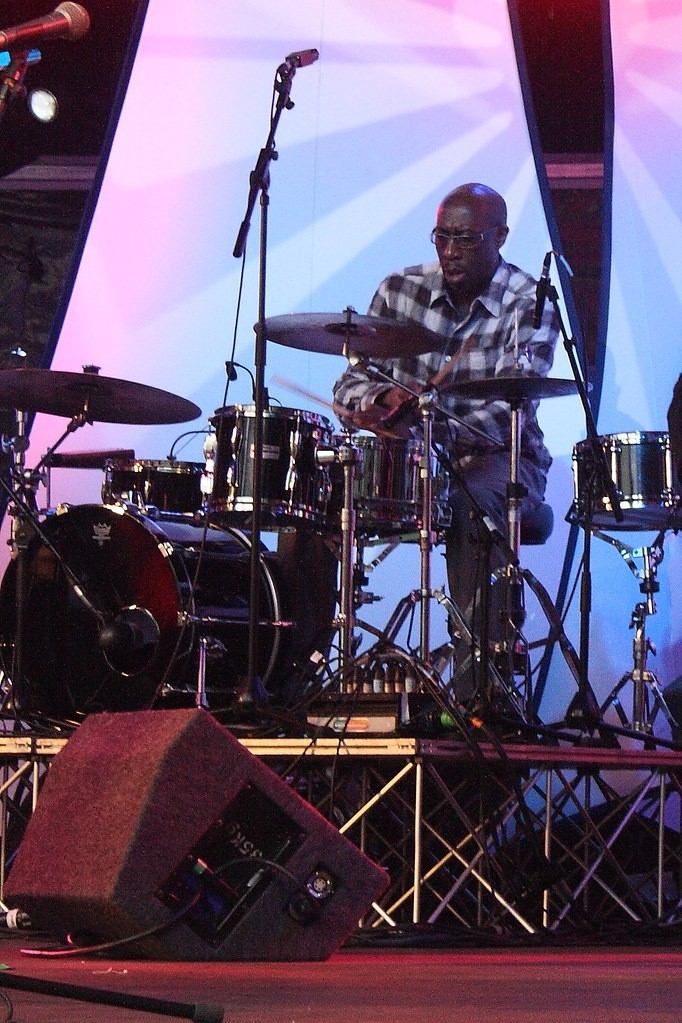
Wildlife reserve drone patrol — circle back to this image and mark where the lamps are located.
[26,64,76,123]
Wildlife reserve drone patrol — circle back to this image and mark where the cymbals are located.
[0,368,202,426]
[253,312,445,358]
[438,370,594,399]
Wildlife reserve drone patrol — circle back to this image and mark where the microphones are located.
[532,252,552,330]
[275,48,319,75]
[225,361,255,399]
[380,381,438,431]
[0,1,91,48]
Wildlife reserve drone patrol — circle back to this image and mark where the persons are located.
[252,182,561,745]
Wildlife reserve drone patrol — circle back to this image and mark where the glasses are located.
[430,226,499,248]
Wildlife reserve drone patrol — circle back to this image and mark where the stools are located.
[447,502,554,705]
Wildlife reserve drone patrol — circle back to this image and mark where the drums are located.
[572,431,682,531]
[0,502,281,728]
[198,404,335,534]
[333,433,455,541]
[103,459,206,515]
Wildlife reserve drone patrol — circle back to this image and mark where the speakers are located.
[1,707,391,962]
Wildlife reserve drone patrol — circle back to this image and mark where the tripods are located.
[206,75,680,751]
